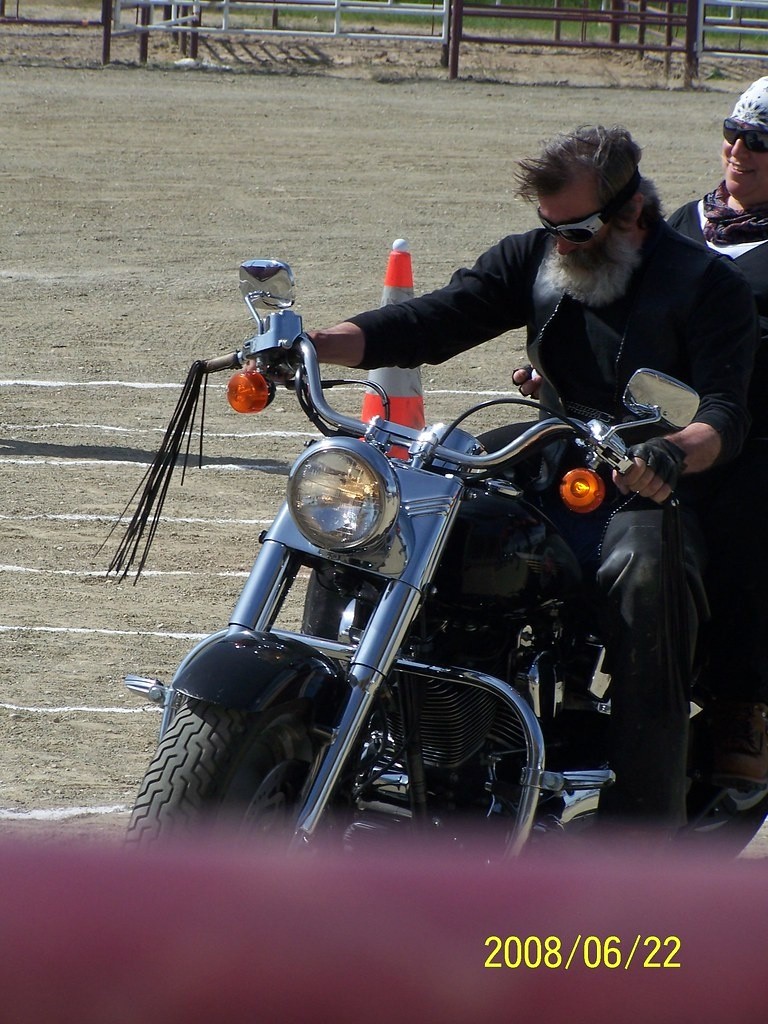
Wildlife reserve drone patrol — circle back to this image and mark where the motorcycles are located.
[123,257,767,875]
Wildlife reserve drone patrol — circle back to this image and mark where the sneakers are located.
[712,702,768,794]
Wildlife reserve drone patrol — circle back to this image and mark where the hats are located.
[730,75,768,131]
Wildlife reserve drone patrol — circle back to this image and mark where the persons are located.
[240,126,760,827]
[510,74,768,791]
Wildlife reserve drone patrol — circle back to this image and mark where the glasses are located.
[537,206,605,245]
[723,119,768,152]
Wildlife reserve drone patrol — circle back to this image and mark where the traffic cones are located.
[355,236,428,462]
